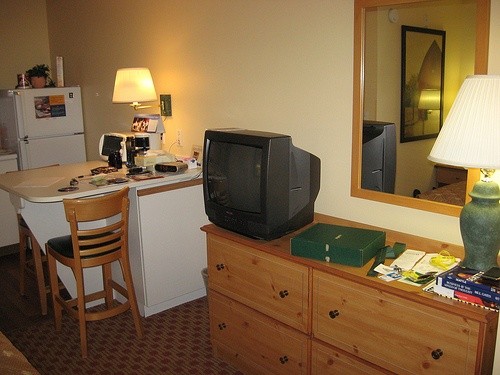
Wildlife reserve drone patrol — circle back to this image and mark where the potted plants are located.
[26,64,50,88]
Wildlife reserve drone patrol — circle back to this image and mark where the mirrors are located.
[351,0,491,218]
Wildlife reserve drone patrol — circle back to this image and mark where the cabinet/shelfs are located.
[0,153,19,257]
[200,212,500,375]
[0,161,213,318]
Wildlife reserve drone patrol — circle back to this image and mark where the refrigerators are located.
[0,86,88,171]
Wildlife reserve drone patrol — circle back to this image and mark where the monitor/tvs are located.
[360,120,397,196]
[203,127,321,241]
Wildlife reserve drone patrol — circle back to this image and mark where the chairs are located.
[8,164,143,358]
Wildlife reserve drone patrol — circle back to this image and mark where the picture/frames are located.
[400,25,446,144]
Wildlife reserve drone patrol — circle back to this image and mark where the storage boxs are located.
[290,222,386,267]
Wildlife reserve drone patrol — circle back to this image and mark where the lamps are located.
[112,67,172,116]
[427,75,500,271]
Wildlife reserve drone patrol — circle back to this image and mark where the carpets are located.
[0,249,243,375]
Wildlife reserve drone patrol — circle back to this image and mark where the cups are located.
[17,73,29,89]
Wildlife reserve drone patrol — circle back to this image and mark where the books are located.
[434,265,500,310]
